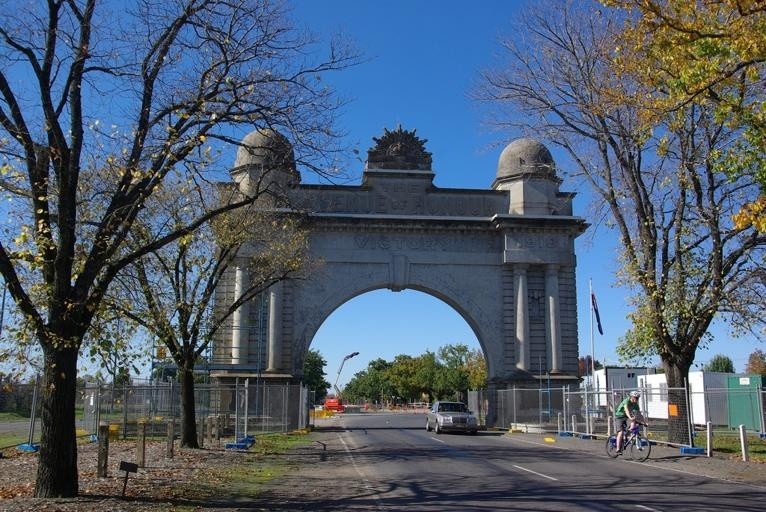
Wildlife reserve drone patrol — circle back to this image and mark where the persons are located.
[614,391,644,455]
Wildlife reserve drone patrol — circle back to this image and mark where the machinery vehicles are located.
[323,352,360,414]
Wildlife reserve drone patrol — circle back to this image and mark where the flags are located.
[591,287,603,335]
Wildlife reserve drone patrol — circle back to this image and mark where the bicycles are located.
[606,411,653,462]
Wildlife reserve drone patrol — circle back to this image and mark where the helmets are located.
[629,391,640,397]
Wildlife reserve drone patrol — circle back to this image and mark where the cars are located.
[425,400,479,434]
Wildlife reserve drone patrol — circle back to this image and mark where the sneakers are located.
[616,450,622,455]
